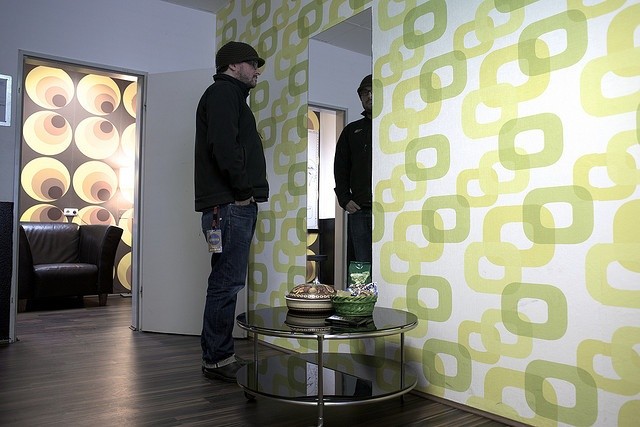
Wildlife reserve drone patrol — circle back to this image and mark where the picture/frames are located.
[0,74,13,127]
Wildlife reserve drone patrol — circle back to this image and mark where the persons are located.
[332,73,371,281]
[192,40,270,382]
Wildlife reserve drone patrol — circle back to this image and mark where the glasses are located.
[359,89,372,96]
[245,61,258,70]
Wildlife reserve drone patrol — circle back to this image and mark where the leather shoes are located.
[204,362,242,383]
[202,355,253,372]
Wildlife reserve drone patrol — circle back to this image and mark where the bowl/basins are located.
[285,295,336,312]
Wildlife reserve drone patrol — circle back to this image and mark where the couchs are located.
[19,222,124,312]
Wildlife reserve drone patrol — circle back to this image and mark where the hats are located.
[357,74,372,101]
[216,41,265,71]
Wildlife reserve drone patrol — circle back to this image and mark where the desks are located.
[236,305,419,426]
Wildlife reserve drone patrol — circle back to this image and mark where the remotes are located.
[326,313,368,327]
